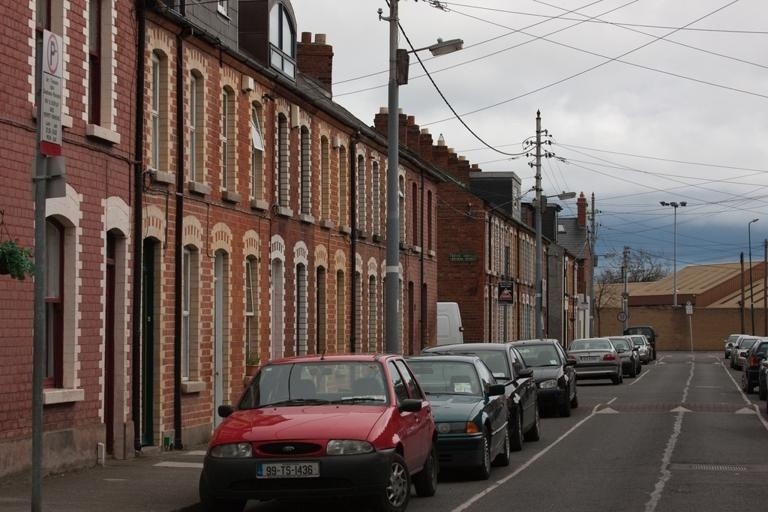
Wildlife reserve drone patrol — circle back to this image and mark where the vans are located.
[623,327,657,360]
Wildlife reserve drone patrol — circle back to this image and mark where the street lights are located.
[661,201,688,306]
[748,217,759,336]
[385,37,464,353]
[535,191,576,338]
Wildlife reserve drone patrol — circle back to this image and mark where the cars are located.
[404,339,578,481]
[724,334,768,399]
[567,335,652,384]
[197,354,439,511]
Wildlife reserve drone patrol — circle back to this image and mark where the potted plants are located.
[0,237,35,281]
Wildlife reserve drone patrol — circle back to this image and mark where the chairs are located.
[616,344,624,349]
[538,350,555,365]
[278,379,317,401]
[353,376,384,399]
[449,376,472,395]
